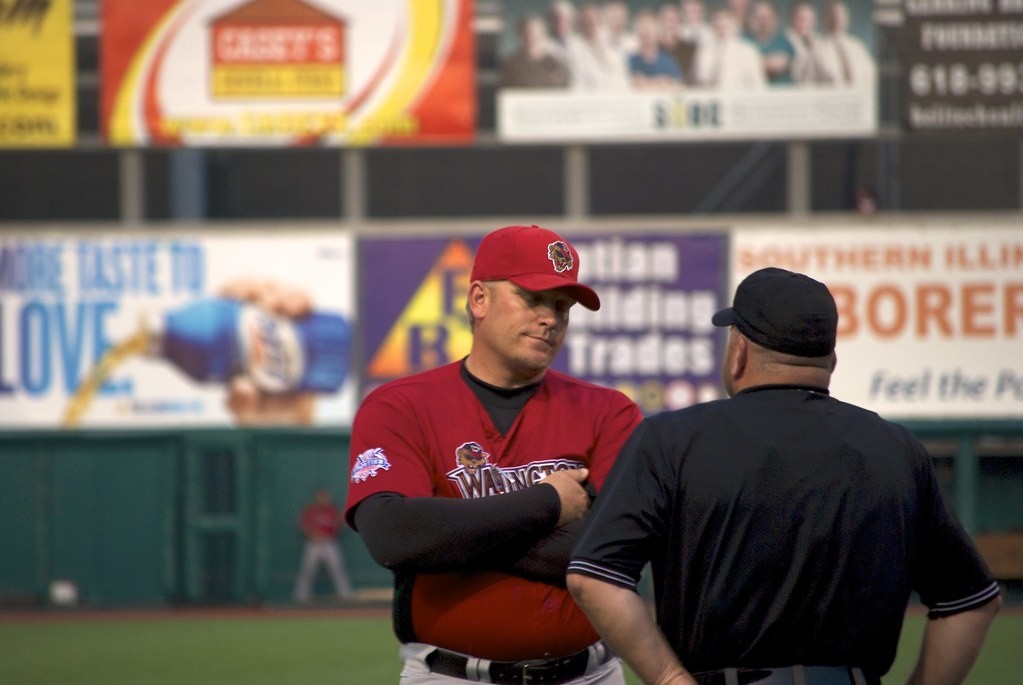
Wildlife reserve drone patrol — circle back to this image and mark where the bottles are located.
[145,297,349,392]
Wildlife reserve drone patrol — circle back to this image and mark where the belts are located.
[426,642,616,685]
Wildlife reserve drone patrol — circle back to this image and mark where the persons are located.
[346,225,646,685]
[511,4,889,89]
[293,492,352,604]
[215,282,319,428]
[567,267,1004,685]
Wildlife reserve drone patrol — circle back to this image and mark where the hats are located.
[469,224,601,311]
[711,266,839,358]
